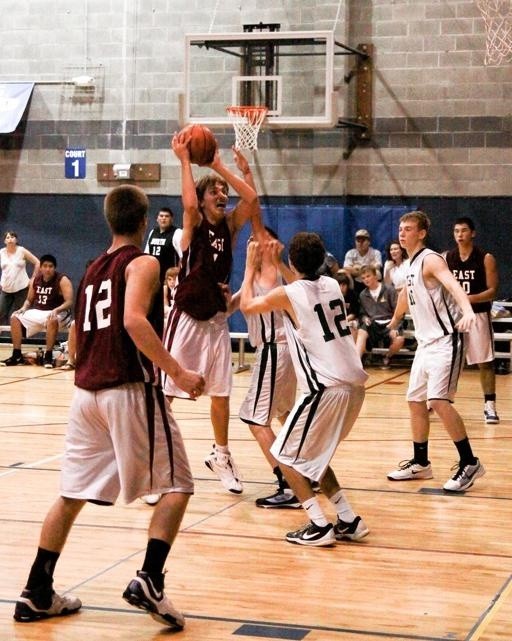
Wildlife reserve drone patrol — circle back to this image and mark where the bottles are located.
[36,347,44,366]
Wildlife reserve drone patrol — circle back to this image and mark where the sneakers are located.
[43,357,54,368]
[381,355,391,371]
[482,400,501,425]
[255,483,303,510]
[332,513,371,542]
[13,588,82,621]
[442,456,486,493]
[205,449,243,495]
[287,519,337,547]
[0,354,24,367]
[122,569,186,629]
[387,456,435,481]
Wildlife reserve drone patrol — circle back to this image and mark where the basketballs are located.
[177,123,215,164]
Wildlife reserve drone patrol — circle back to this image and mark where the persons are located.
[159,128,257,498]
[343,229,383,292]
[358,264,404,371]
[144,207,184,282]
[58,320,76,372]
[229,146,327,511]
[383,240,410,295]
[163,267,180,330]
[239,230,371,547]
[384,211,486,494]
[0,254,74,369]
[0,231,40,314]
[440,218,501,426]
[332,271,360,343]
[13,186,198,631]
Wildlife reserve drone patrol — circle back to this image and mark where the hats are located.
[354,228,370,239]
